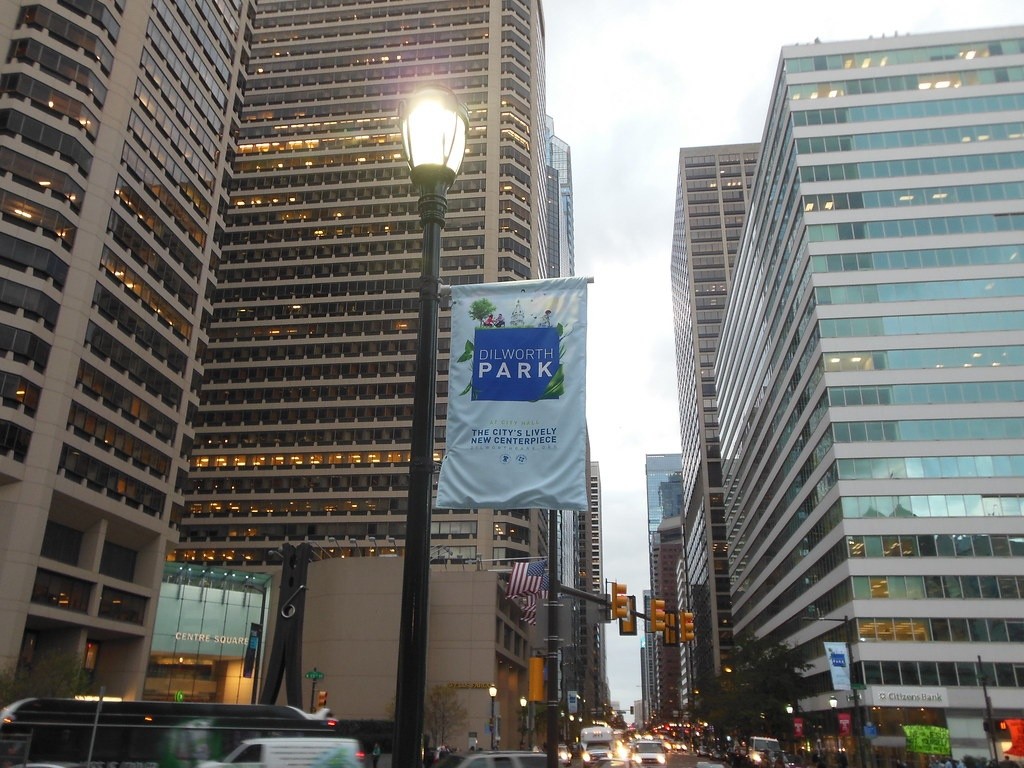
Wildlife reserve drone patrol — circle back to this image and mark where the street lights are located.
[829,694,839,752]
[488,682,498,751]
[519,696,527,750]
[786,703,796,756]
[384,87,471,768]
[800,613,866,767]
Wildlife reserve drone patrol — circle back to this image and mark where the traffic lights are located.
[318,691,328,714]
[681,613,699,643]
[649,597,666,633]
[983,720,989,733]
[997,720,1008,733]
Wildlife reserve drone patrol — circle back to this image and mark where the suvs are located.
[629,740,668,768]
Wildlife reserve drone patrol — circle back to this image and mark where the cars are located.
[0,696,340,768]
[559,725,628,768]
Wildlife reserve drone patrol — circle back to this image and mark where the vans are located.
[432,751,559,767]
[217,737,364,768]
[749,734,781,768]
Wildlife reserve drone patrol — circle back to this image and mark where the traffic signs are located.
[612,580,629,620]
